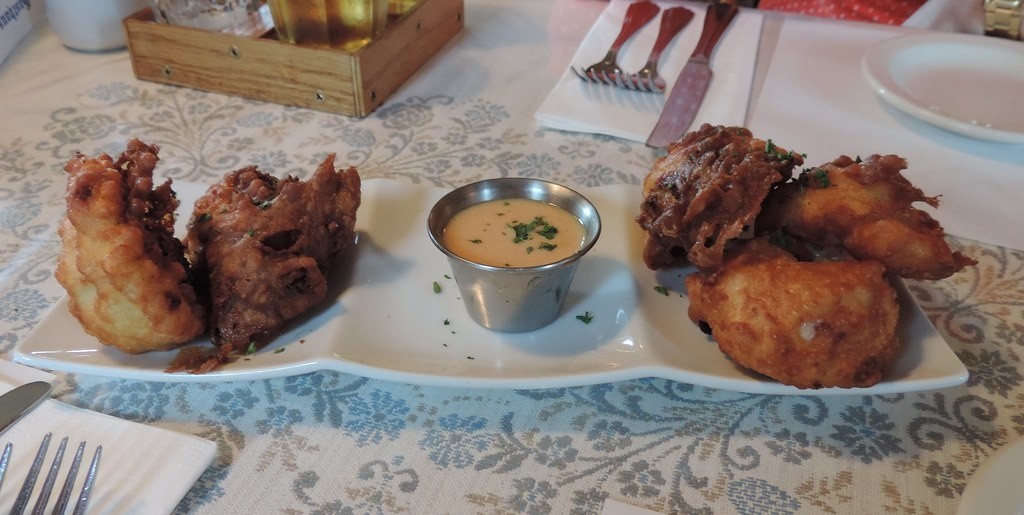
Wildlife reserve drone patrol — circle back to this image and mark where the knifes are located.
[645,2,739,148]
[0,380,52,435]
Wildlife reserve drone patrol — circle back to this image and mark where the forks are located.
[614,6,695,93]
[571,0,660,87]
[8,432,103,515]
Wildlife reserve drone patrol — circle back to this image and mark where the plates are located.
[860,31,1024,144]
[13,183,968,397]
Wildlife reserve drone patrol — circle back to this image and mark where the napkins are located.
[0,356,220,515]
[535,1,1024,253]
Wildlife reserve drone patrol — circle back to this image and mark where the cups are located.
[266,0,388,54]
[388,0,421,26]
[45,0,149,52]
[426,177,602,332]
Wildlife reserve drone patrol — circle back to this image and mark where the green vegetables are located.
[171,129,862,361]
